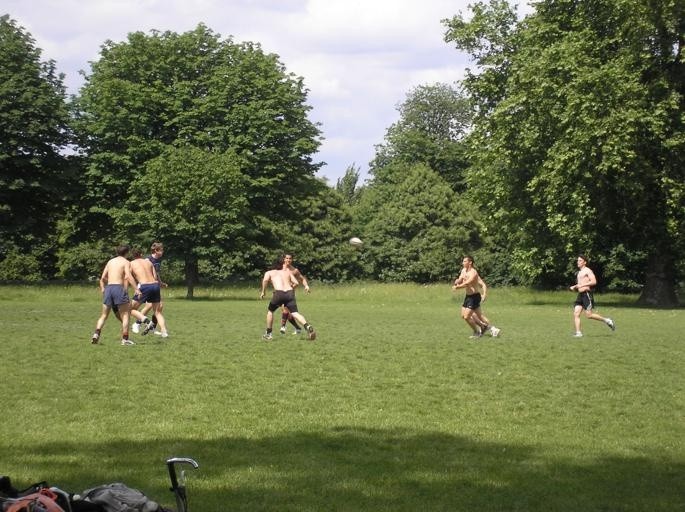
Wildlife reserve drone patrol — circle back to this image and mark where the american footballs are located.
[350,237,364,246]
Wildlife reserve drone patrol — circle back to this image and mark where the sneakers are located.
[122,317,168,346]
[91,334,99,343]
[263,325,315,341]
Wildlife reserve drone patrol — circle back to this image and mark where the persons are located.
[453,256,488,337]
[280,254,311,334]
[261,256,316,340]
[468,275,501,338]
[129,249,167,337]
[570,254,615,337]
[91,245,134,345]
[132,242,168,334]
